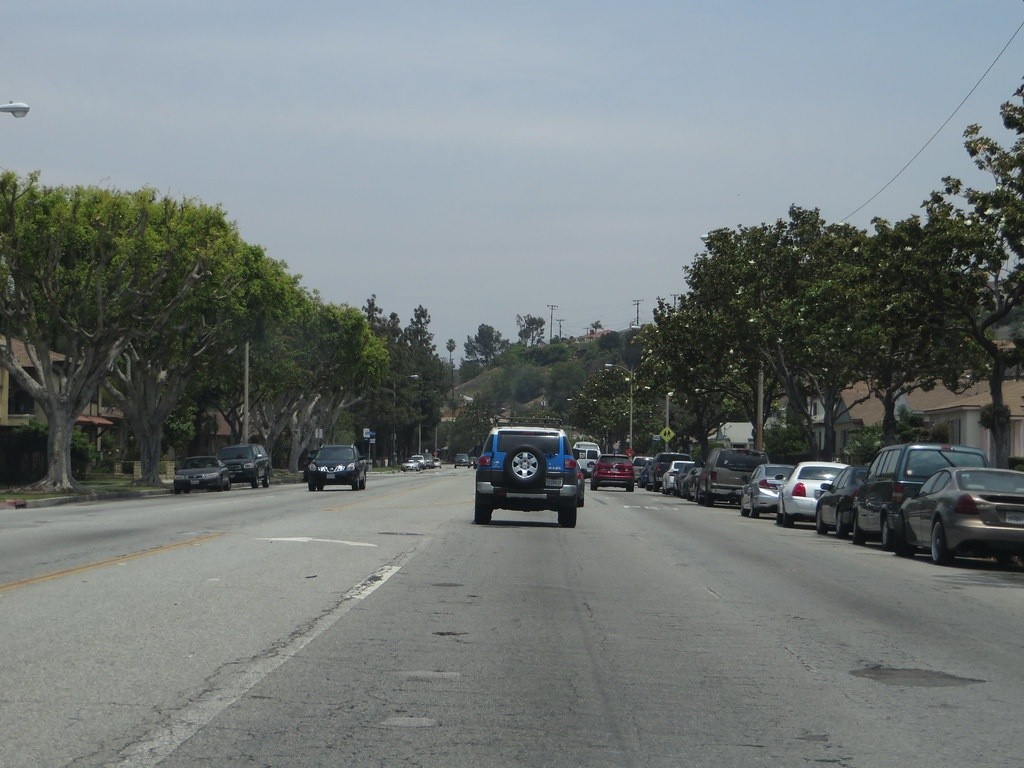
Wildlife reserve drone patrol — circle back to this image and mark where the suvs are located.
[305,443,367,491]
[217,444,272,489]
[852,441,994,553]
[693,446,771,507]
[453,453,471,469]
[473,416,580,528]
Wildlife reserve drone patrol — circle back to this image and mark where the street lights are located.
[700,234,766,451]
[391,374,419,468]
[629,325,670,452]
[606,363,635,461]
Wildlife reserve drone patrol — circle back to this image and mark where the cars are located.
[774,460,850,527]
[572,440,706,508]
[739,463,797,519]
[893,467,1024,570]
[401,453,442,472]
[814,464,872,539]
[172,456,232,495]
[470,456,478,466]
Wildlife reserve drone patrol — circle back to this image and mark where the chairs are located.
[1015,488,1024,495]
[966,484,984,491]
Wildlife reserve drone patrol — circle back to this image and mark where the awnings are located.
[88,417,113,425]
[77,415,95,424]
